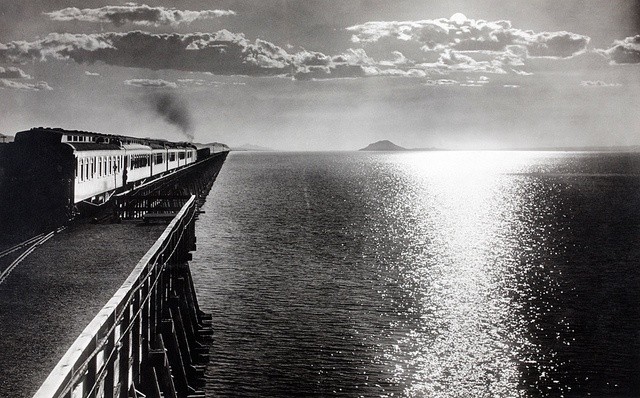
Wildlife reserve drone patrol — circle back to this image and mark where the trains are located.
[8,126,197,208]
